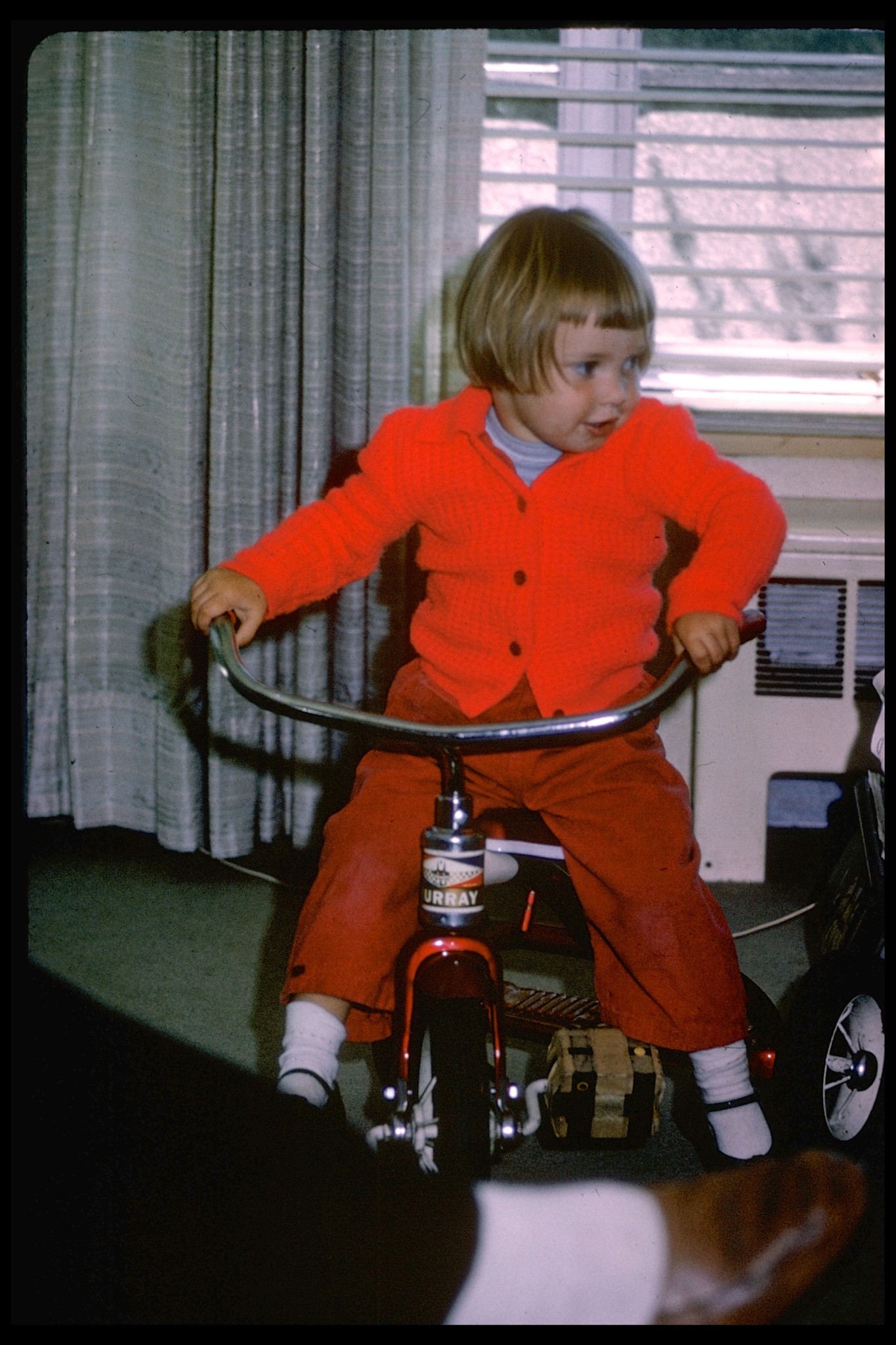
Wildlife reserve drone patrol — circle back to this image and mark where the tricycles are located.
[201,606,895,1185]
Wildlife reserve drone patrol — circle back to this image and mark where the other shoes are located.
[696,1077,776,1167]
[279,1068,346,1112]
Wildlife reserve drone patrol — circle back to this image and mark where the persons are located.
[189,200,798,1172]
